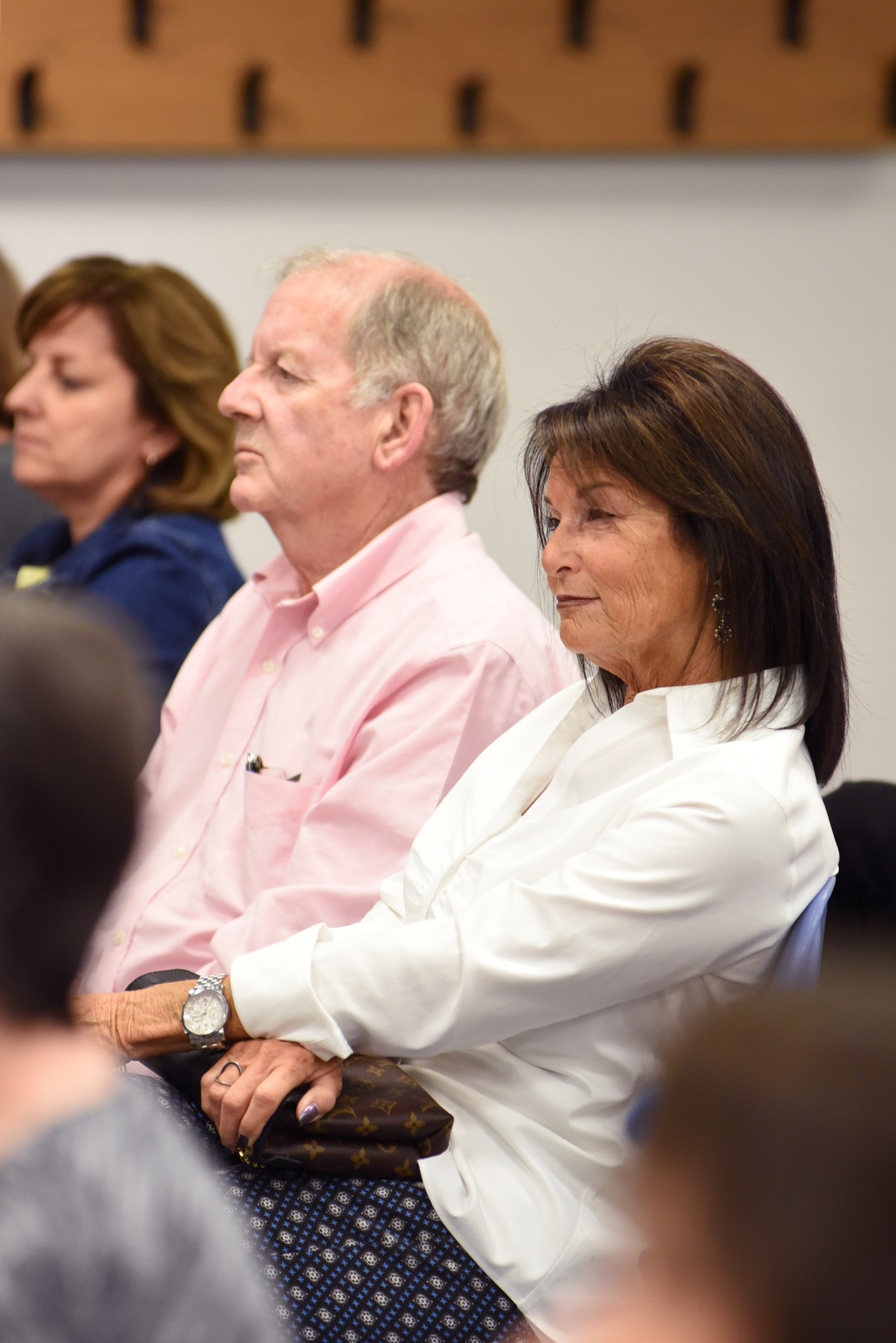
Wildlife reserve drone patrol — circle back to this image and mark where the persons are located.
[0,260,61,584]
[0,584,285,1343]
[625,956,895,1343]
[1,248,252,719]
[66,337,851,1341]
[63,249,579,1081]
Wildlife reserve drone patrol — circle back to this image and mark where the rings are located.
[214,1062,243,1087]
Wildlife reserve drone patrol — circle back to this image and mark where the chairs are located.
[818,781,895,978]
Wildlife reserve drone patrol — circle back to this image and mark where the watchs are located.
[179,974,230,1053]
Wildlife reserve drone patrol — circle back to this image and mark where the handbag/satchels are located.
[120,969,456,1180]
